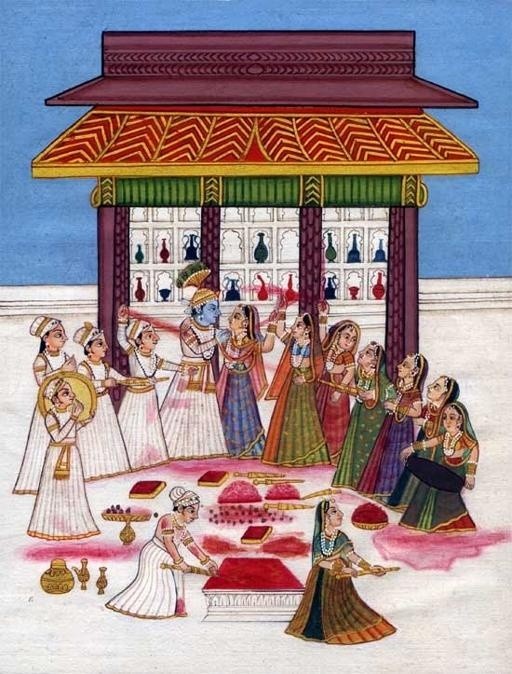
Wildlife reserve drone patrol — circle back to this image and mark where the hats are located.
[42,377,65,400]
[177,263,217,305]
[169,485,200,507]
[73,322,105,346]
[29,316,62,337]
[125,320,153,340]
